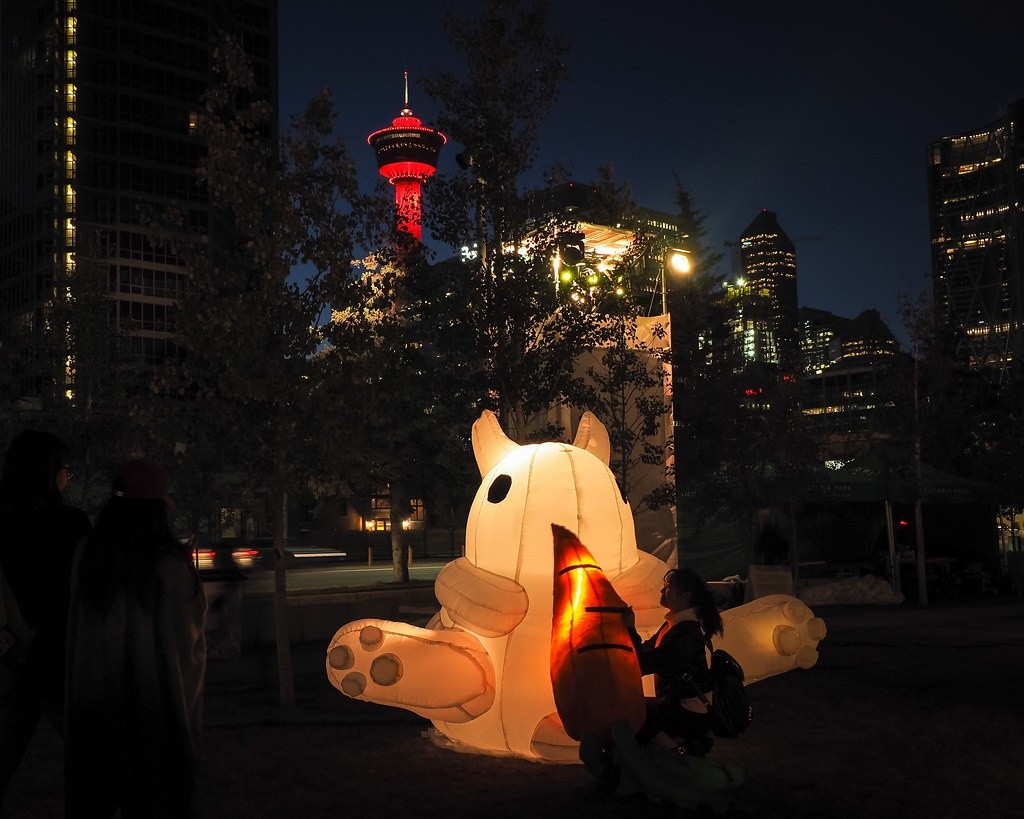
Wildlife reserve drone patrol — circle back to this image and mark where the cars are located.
[179,537,257,574]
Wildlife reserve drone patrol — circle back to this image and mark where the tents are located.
[769,443,1009,604]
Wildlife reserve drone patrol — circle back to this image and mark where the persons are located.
[621,567,725,773]
[62,460,208,818]
[0,430,90,803]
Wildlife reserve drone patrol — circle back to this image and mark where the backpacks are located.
[670,624,754,739]
